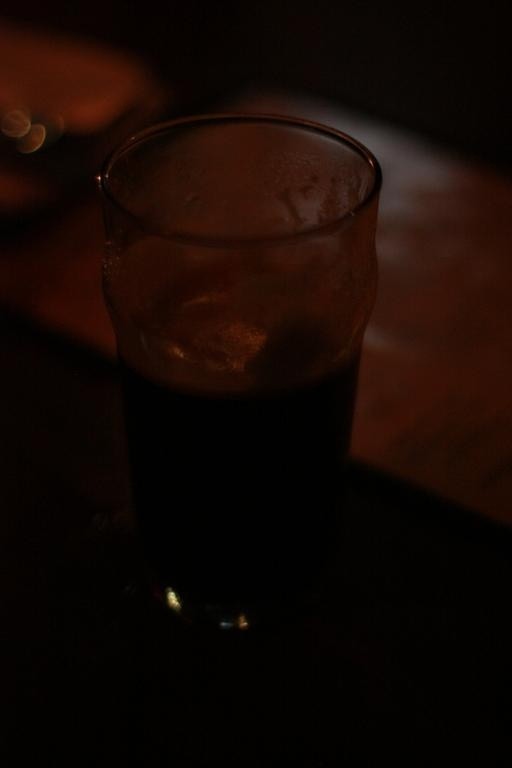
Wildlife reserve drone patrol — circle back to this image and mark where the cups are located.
[91,108,386,634]
